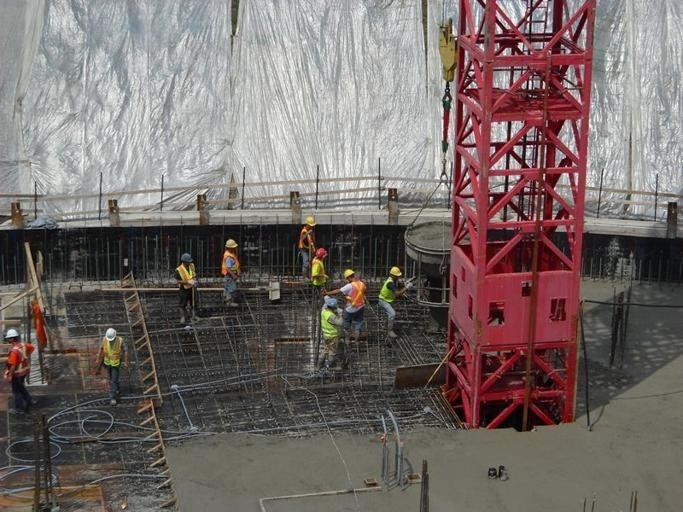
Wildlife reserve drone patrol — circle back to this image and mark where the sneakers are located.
[110,398,117,405]
[180,316,185,324]
[329,366,342,371]
[8,409,23,417]
[388,329,397,338]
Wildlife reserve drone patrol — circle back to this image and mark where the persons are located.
[94,328,128,406]
[2,328,34,410]
[219,239,241,306]
[309,248,413,370]
[298,217,316,279]
[173,251,199,323]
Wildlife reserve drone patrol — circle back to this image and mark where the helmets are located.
[327,298,338,307]
[316,247,327,257]
[344,269,355,279]
[105,327,117,341]
[305,217,316,226]
[390,266,402,276]
[225,239,239,248]
[181,253,194,263]
[5,329,20,338]
